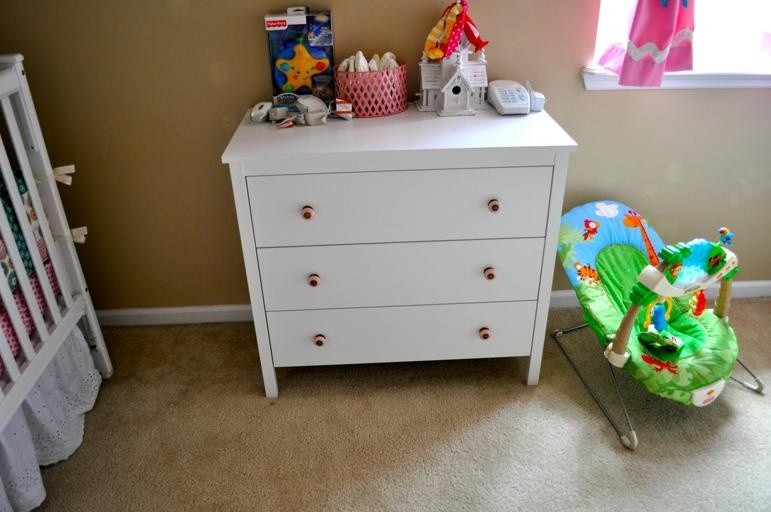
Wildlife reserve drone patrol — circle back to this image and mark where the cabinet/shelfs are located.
[218,99,583,401]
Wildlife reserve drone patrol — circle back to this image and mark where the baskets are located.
[333,62,408,119]
